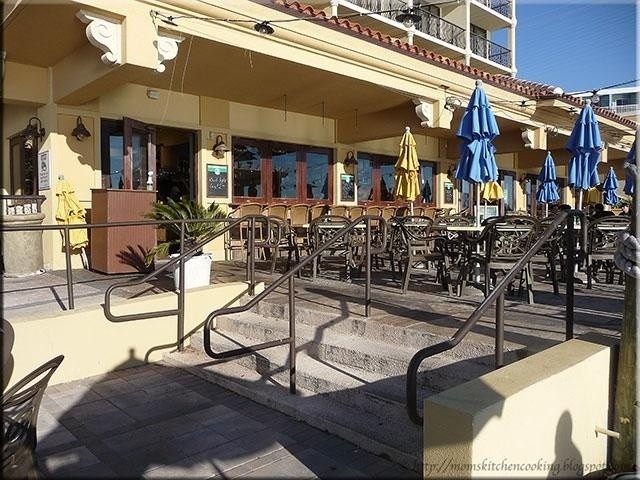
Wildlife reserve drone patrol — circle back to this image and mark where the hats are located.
[619,202,629,208]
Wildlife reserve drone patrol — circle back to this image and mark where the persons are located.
[618,202,632,222]
[210,133,225,159]
[341,151,358,175]
[590,203,616,235]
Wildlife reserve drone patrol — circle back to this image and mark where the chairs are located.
[0,353,67,480]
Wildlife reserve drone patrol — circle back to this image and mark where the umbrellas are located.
[622,130,636,201]
[455,78,501,283]
[563,98,603,274]
[534,150,561,219]
[603,165,620,208]
[392,125,421,256]
[53,175,91,273]
[478,183,505,221]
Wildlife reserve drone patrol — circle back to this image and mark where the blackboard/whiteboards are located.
[205,163,229,199]
[474,205,499,227]
[443,181,455,206]
[338,173,356,203]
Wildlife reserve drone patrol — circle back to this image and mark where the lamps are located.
[253,21,276,35]
[21,116,47,141]
[343,150,358,167]
[396,7,422,29]
[72,114,91,142]
[213,135,230,153]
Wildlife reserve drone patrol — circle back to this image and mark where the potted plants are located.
[144,196,228,293]
